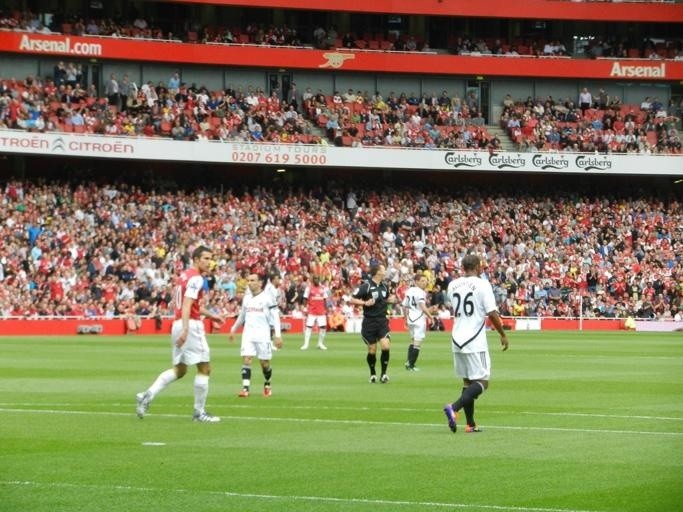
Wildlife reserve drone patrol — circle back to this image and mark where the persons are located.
[136,246,226,423]
[447,254,509,433]
[349,264,395,384]
[402,274,436,372]
[227,272,282,397]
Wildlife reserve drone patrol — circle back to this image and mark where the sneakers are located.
[404,361,419,373]
[134,391,150,419]
[380,374,389,384]
[237,387,250,398]
[299,343,328,350]
[464,422,483,433]
[191,411,221,423]
[262,385,273,398]
[369,374,377,385]
[442,403,460,433]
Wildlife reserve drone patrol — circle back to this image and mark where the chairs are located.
[586,40,680,61]
[184,24,437,54]
[454,34,571,58]
[2,1,183,43]
[303,95,680,155]
[0,81,335,146]
